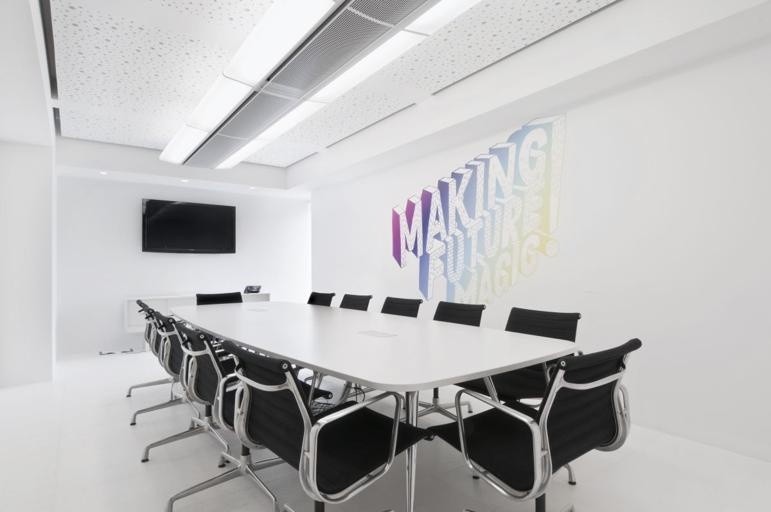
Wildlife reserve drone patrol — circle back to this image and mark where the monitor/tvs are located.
[141,198,236,253]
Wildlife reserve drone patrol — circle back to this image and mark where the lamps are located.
[157,0,485,169]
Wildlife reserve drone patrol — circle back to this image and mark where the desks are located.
[172,299,580,512]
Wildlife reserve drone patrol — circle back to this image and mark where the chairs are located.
[341,294,373,311]
[308,291,335,307]
[196,292,244,305]
[380,296,423,318]
[435,339,641,511]
[128,299,333,463]
[222,339,434,512]
[167,323,332,512]
[433,301,485,327]
[435,307,581,486]
